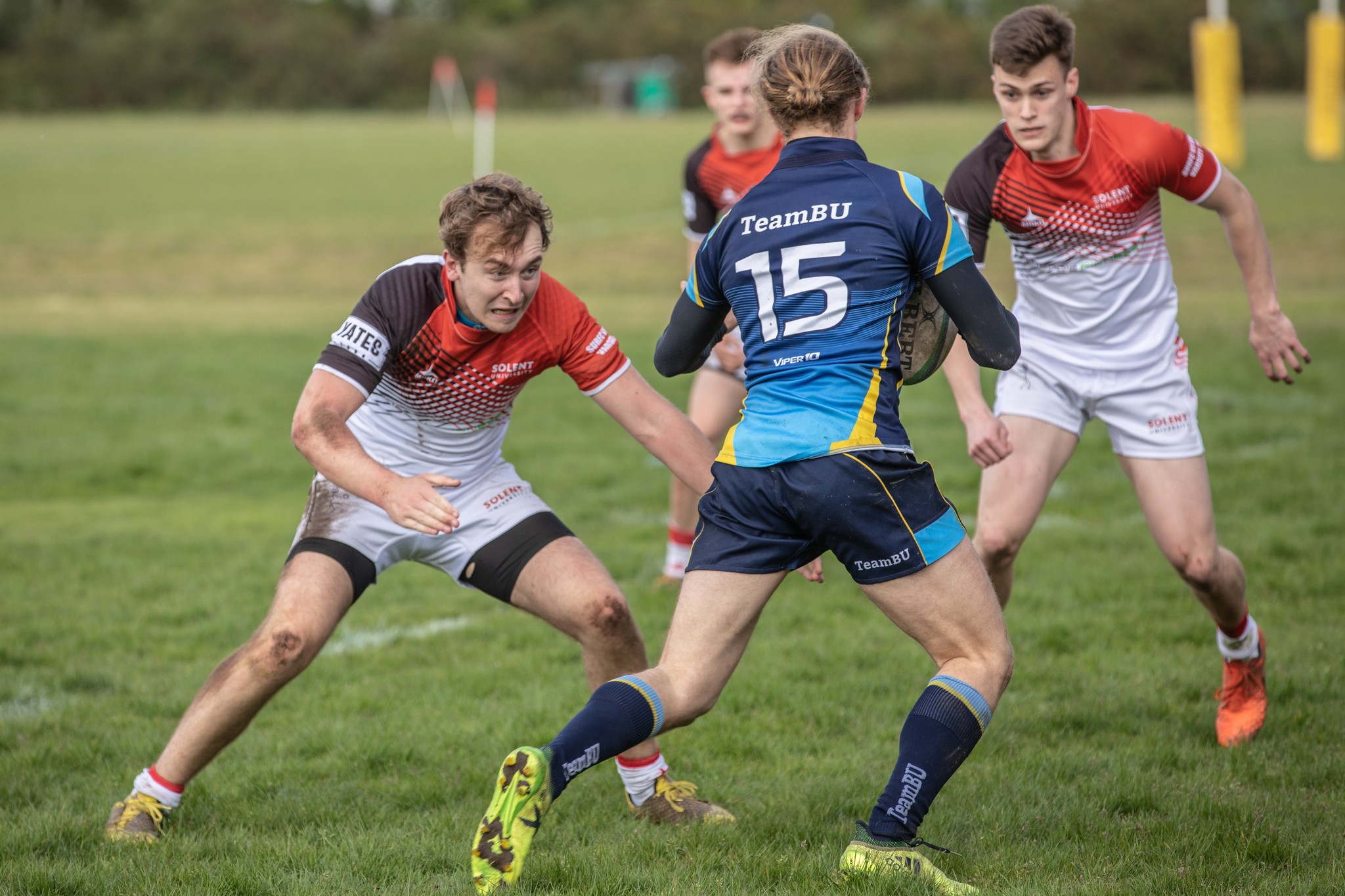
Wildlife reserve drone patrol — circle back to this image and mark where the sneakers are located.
[839,818,979,896]
[1214,631,1267,747]
[623,774,736,825]
[469,745,562,896]
[105,792,173,848]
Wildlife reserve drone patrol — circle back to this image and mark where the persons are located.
[944,4,1313,747]
[104,175,824,841]
[660,31,789,584]
[470,23,1020,895]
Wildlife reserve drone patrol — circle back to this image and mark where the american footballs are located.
[897,269,961,386]
